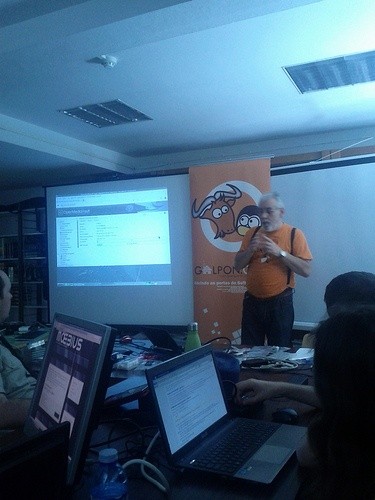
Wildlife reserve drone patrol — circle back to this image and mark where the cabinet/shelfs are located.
[0,196,51,324]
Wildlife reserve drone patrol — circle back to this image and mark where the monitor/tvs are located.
[0,311,118,500]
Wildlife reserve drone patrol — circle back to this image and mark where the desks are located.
[5,343,315,500]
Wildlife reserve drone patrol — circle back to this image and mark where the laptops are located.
[144,341,310,490]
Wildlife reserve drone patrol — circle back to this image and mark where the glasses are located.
[257,207,280,215]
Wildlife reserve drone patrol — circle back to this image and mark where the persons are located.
[0,269,38,428]
[234,193,313,347]
[230,271,375,500]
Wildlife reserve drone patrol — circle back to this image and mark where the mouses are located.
[271,406,306,426]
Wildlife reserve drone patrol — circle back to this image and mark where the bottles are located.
[86,448,130,499]
[186,322,201,352]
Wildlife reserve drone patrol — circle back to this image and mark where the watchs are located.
[278,249,286,259]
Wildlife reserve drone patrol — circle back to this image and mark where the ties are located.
[0,332,37,379]
